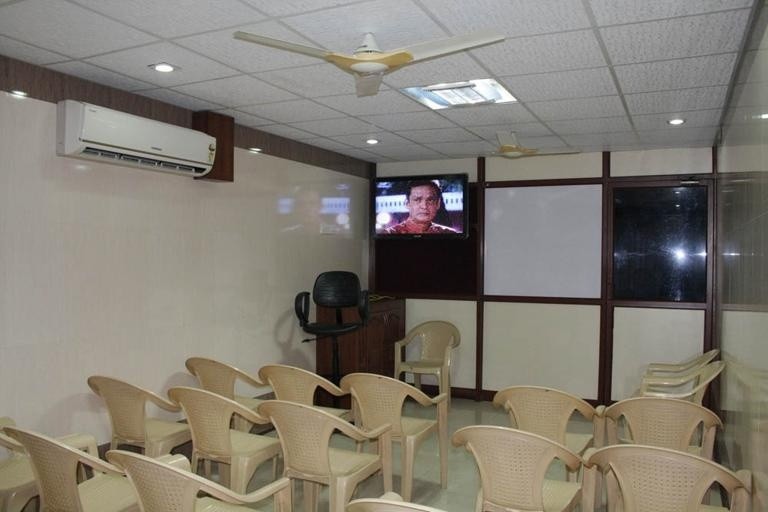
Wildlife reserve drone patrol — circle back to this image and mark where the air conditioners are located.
[56,99,216,178]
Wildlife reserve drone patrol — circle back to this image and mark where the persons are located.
[381,181,457,233]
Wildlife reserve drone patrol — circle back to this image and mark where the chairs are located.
[632,361,728,406]
[167,386,282,496]
[630,349,721,401]
[590,445,753,512]
[87,375,193,458]
[0,416,103,512]
[605,398,725,505]
[341,373,447,504]
[452,425,595,512]
[394,321,461,408]
[184,356,264,433]
[257,400,393,512]
[346,492,447,512]
[104,449,292,512]
[258,365,362,438]
[493,386,605,483]
[294,270,370,434]
[3,426,172,512]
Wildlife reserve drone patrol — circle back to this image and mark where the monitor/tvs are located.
[369,173,469,241]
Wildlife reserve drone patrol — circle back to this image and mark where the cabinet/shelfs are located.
[316,299,406,409]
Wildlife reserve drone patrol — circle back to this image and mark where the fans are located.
[231,31,506,98]
[449,131,582,159]
